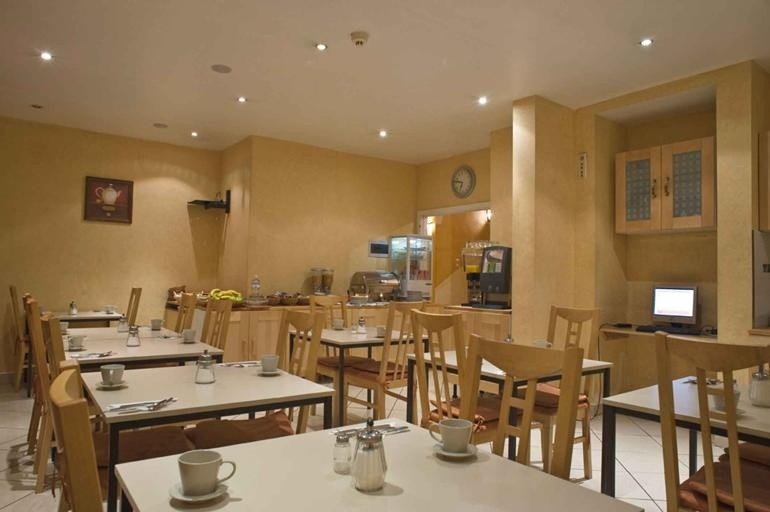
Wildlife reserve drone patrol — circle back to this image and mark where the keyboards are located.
[636,325,700,335]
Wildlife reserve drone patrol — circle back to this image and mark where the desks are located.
[116,416,645,512]
[599,323,769,408]
[80,361,336,512]
[603,363,770,497]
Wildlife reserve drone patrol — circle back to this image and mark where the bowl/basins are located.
[350,296,367,305]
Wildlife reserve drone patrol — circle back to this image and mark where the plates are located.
[431,441,476,460]
[167,483,230,504]
[256,368,281,376]
[95,382,129,389]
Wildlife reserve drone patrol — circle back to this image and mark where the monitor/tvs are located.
[651,285,698,327]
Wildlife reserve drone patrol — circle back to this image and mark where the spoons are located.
[109,394,178,417]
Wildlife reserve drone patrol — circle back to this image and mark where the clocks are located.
[451,164,476,199]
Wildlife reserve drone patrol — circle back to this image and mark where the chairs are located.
[49,370,105,511]
[8,286,613,493]
[654,330,770,511]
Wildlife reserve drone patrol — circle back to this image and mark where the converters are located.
[615,323,632,328]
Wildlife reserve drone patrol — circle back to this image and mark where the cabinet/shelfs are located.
[389,234,434,299]
[614,134,717,235]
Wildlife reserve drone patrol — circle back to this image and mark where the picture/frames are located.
[84,175,134,223]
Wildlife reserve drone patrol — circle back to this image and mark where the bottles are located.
[117,312,130,333]
[747,365,770,407]
[357,316,367,334]
[332,416,390,492]
[250,273,260,297]
[124,323,141,347]
[194,349,216,384]
[68,300,78,315]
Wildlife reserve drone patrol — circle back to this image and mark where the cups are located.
[178,450,236,496]
[105,305,114,314]
[463,239,499,248]
[100,363,126,386]
[182,329,196,345]
[427,417,475,453]
[67,335,85,352]
[376,324,388,339]
[260,354,279,372]
[712,388,741,413]
[151,319,166,331]
[59,321,69,336]
[333,319,345,330]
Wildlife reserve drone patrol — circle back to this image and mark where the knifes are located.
[333,423,411,437]
